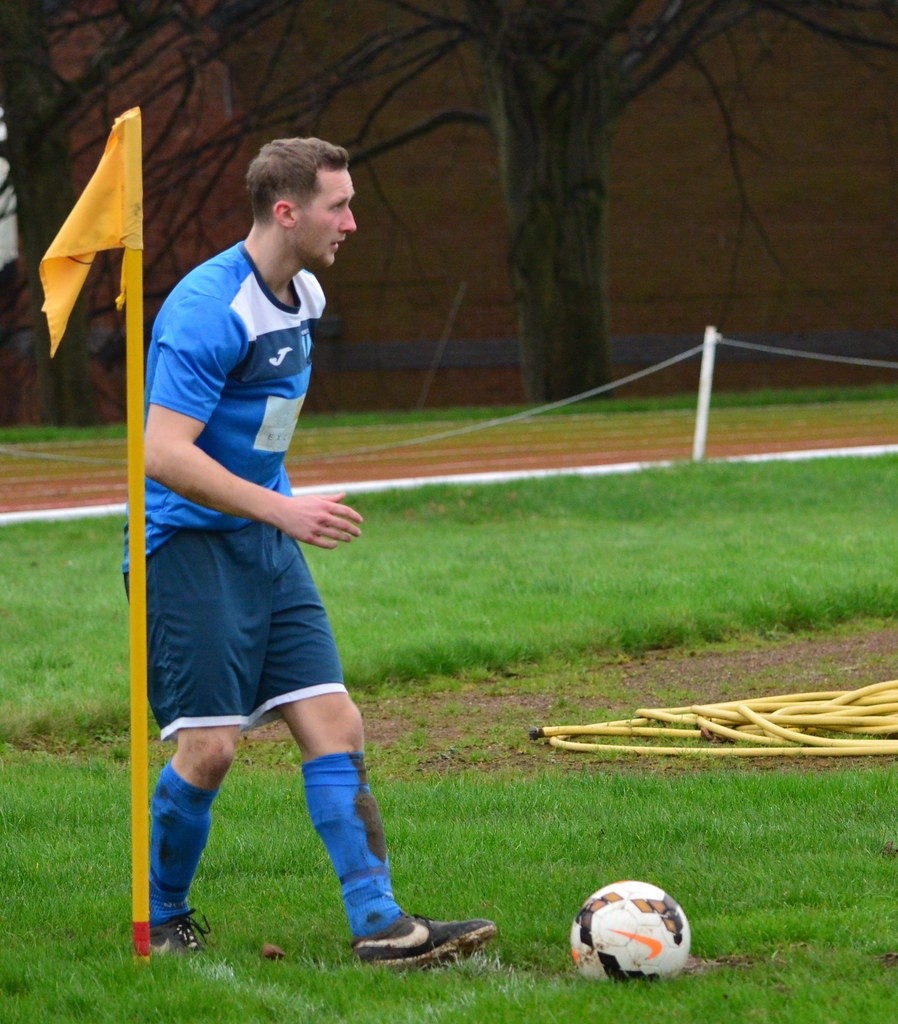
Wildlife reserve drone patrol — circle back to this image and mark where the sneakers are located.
[351,910,496,972]
[150,908,212,954]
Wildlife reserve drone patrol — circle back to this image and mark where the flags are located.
[38,117,125,358]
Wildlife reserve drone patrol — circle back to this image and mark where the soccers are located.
[569,878,691,983]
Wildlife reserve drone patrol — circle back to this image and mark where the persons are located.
[122,137,498,970]
